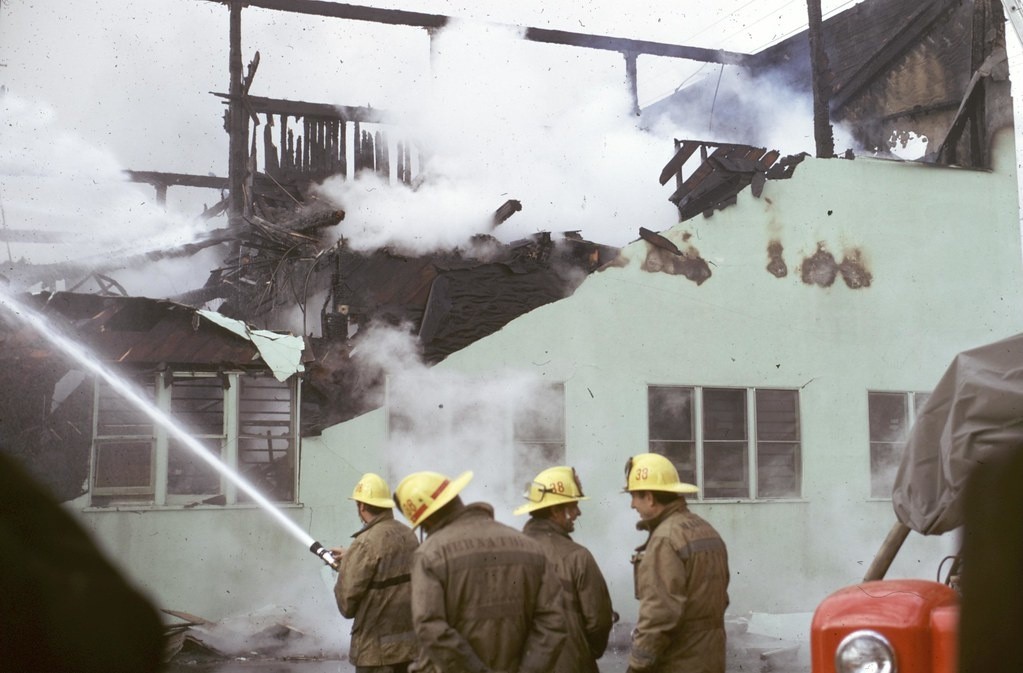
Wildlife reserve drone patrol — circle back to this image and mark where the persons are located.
[622,453,730,673]
[330,474,420,673]
[514,466,613,673]
[393,470,567,673]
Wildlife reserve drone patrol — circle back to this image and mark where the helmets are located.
[392,471,473,531]
[512,466,590,515]
[347,472,395,509]
[620,452,701,494]
[465,502,494,518]
